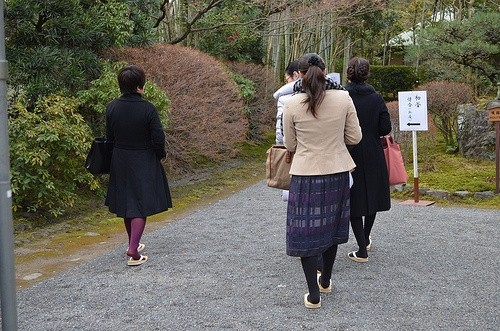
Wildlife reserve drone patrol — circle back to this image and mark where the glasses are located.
[284,73,295,84]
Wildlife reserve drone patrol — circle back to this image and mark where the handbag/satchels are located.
[85,137,110,176]
[266,144,295,190]
[384,136,407,184]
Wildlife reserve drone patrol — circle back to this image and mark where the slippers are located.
[317,274,331,292]
[127,244,145,253]
[128,256,147,266]
[303,292,321,307]
[347,250,368,262]
[366,236,372,251]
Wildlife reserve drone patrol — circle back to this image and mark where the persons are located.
[105,65,173,265]
[342,57,392,263]
[273,60,305,201]
[282,53,363,308]
